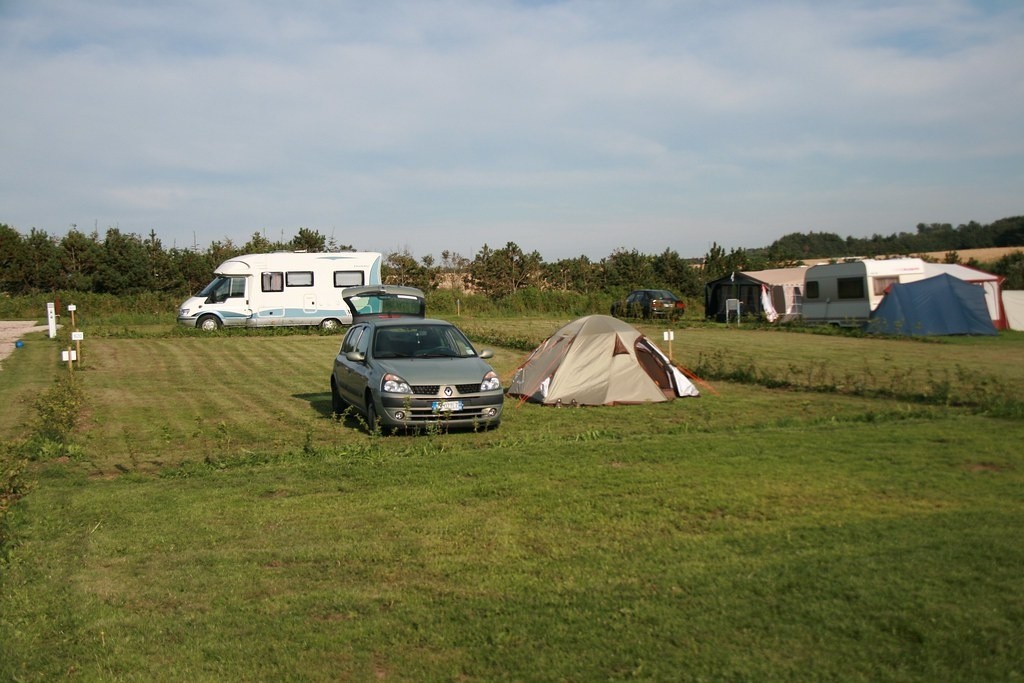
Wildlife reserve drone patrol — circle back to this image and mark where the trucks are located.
[177,250,382,332]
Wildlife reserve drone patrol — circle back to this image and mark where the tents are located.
[507,315,701,406]
[865,263,1011,337]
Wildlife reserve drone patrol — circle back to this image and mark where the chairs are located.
[417,335,438,351]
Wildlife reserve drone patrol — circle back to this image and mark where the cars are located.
[330,283,504,436]
[609,289,685,321]
